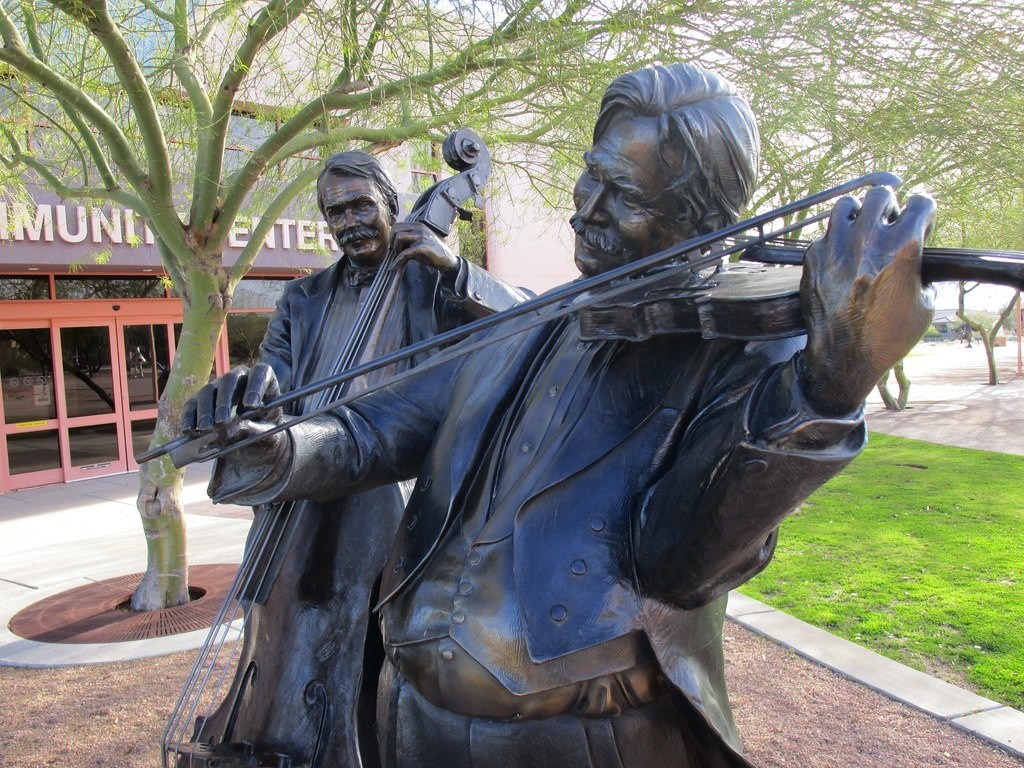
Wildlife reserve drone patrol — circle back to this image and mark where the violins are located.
[576,231,1024,343]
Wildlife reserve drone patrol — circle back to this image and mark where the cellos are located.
[159,127,494,768]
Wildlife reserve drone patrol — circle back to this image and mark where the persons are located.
[251,152,539,514]
[132,346,146,378]
[181,63,937,768]
